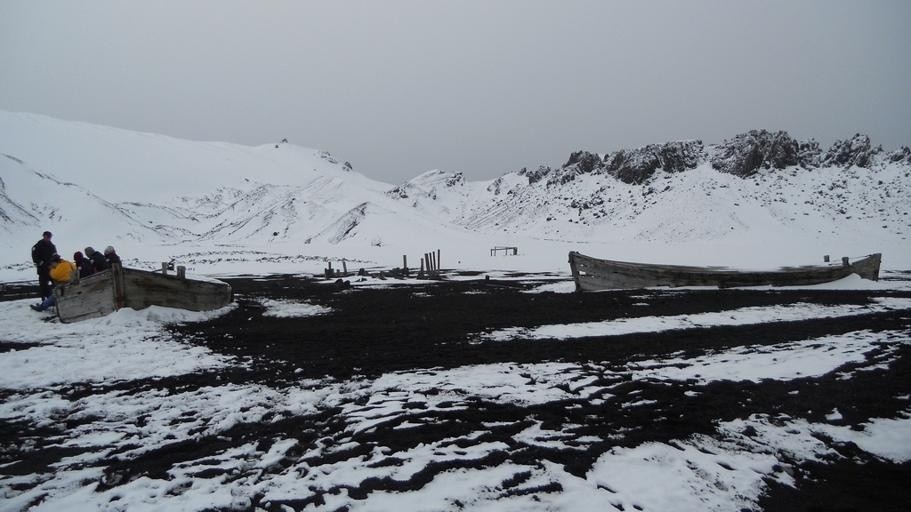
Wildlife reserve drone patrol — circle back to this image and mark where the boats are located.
[567,249,884,293]
[52,262,233,326]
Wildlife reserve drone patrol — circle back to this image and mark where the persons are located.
[30,230,120,313]
